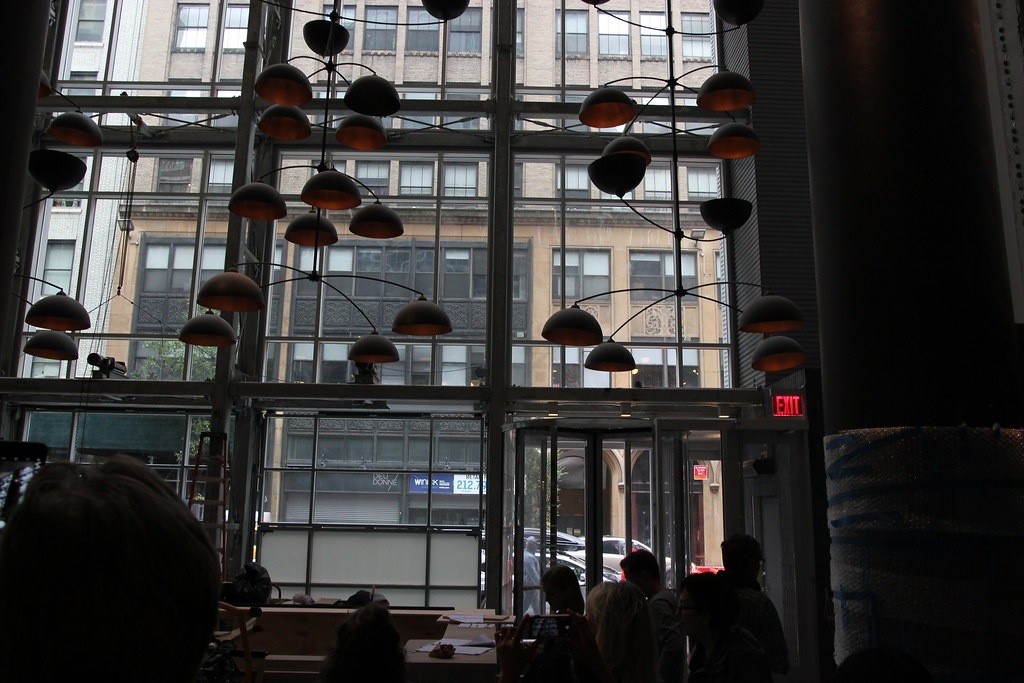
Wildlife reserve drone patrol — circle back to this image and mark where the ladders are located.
[187,431,227,582]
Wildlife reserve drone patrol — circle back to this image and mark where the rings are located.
[512,637,521,640]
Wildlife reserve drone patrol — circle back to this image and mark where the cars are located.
[481,525,697,616]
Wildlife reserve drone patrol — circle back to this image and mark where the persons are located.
[494,534,791,683]
[831,646,945,683]
[314,605,407,683]
[0,455,220,683]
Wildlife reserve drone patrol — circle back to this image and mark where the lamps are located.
[0,0,808,371]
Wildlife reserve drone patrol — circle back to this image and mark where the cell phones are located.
[522,614,572,638]
